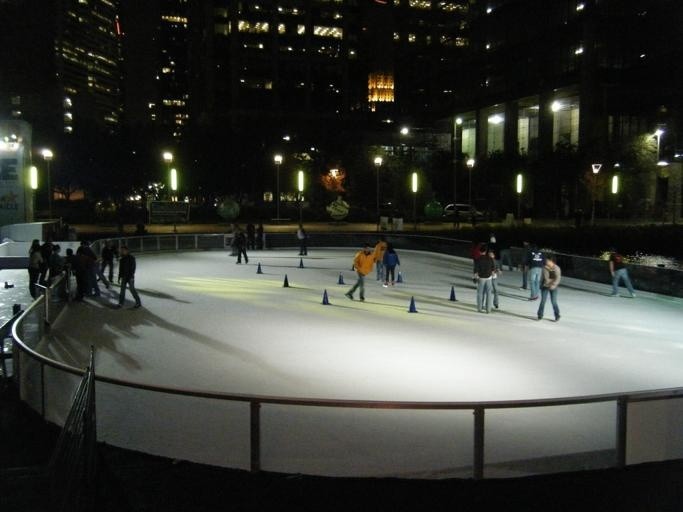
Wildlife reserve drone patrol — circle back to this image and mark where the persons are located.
[383,245,400,285]
[246,220,255,249]
[297,224,308,255]
[472,240,483,282]
[520,242,533,289]
[374,242,387,279]
[118,246,141,308]
[345,244,374,301]
[97,240,119,283]
[489,236,501,273]
[527,245,544,300]
[606,251,637,299]
[474,243,495,313]
[230,222,248,263]
[29,236,110,301]
[482,252,500,308]
[538,255,561,320]
[257,223,263,249]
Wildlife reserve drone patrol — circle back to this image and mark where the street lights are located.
[465,157,476,216]
[168,165,179,202]
[590,161,602,224]
[410,171,418,232]
[295,169,305,228]
[452,116,464,203]
[272,153,284,219]
[373,156,383,224]
[161,150,173,201]
[40,146,54,218]
[30,164,38,223]
[514,172,524,228]
[654,126,664,162]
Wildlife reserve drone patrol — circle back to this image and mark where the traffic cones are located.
[297,256,306,269]
[320,288,330,306]
[446,285,458,303]
[282,273,290,288]
[254,261,263,275]
[335,271,347,285]
[405,294,418,313]
[394,270,403,283]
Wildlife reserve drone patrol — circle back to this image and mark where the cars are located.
[94,200,117,220]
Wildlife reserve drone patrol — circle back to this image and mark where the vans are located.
[442,203,482,218]
